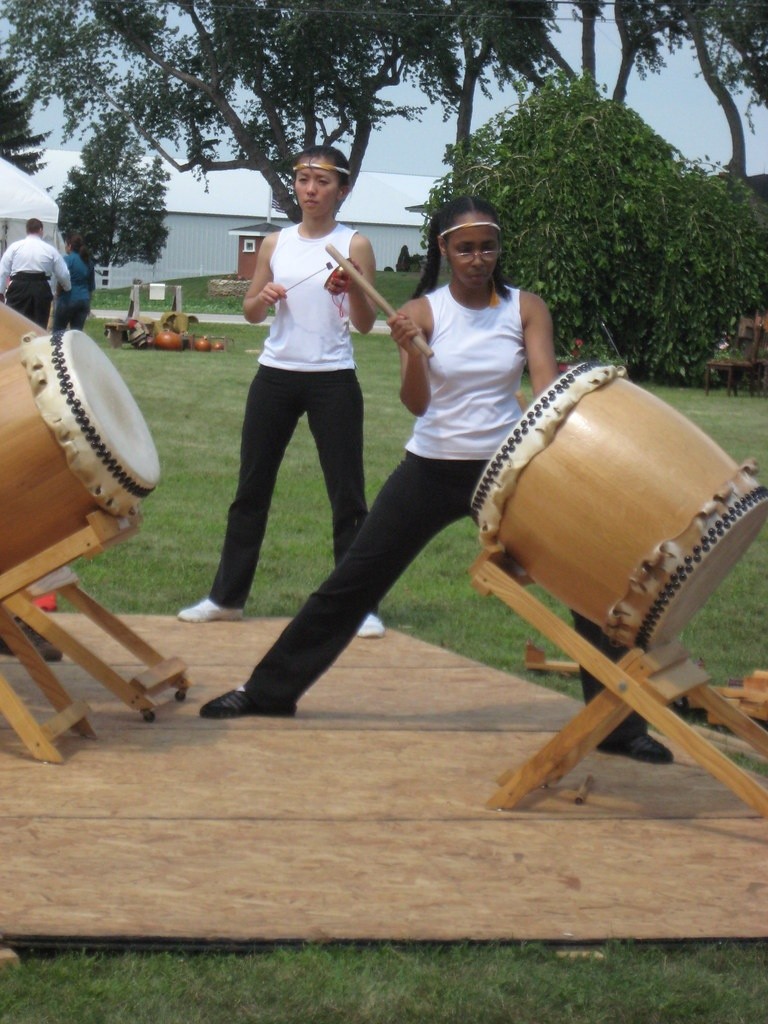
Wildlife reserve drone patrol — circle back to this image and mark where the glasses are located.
[448,235,504,264]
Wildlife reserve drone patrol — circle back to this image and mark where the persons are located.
[199,194,676,765]
[0,218,96,333]
[177,146,386,637]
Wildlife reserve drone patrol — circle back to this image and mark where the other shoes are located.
[199,688,298,719]
[596,732,675,765]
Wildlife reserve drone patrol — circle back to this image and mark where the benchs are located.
[104,322,154,348]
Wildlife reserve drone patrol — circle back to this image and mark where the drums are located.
[1,330,163,580]
[0,300,47,357]
[465,361,768,657]
[323,257,364,296]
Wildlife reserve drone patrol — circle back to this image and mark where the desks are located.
[705,360,753,398]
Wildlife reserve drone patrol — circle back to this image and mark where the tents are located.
[0,157,59,296]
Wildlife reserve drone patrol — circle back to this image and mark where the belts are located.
[11,275,50,281]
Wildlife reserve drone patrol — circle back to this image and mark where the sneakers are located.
[356,614,386,638]
[176,597,245,624]
